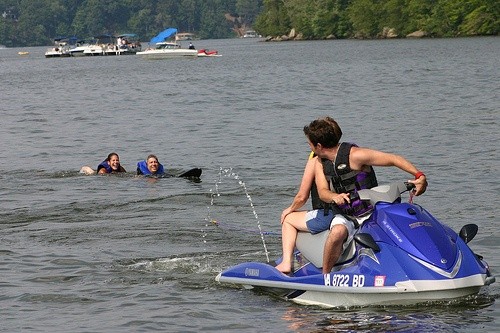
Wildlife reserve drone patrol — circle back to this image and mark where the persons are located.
[116,37,127,45]
[303,116,428,274]
[188,42,195,49]
[80,152,127,175]
[274,150,336,273]
[136,154,165,177]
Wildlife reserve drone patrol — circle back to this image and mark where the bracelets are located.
[415,172,425,180]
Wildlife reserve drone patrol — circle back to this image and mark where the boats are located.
[135,28,198,56]
[45,36,74,57]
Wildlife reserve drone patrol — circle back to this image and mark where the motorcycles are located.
[214,180,496,310]
